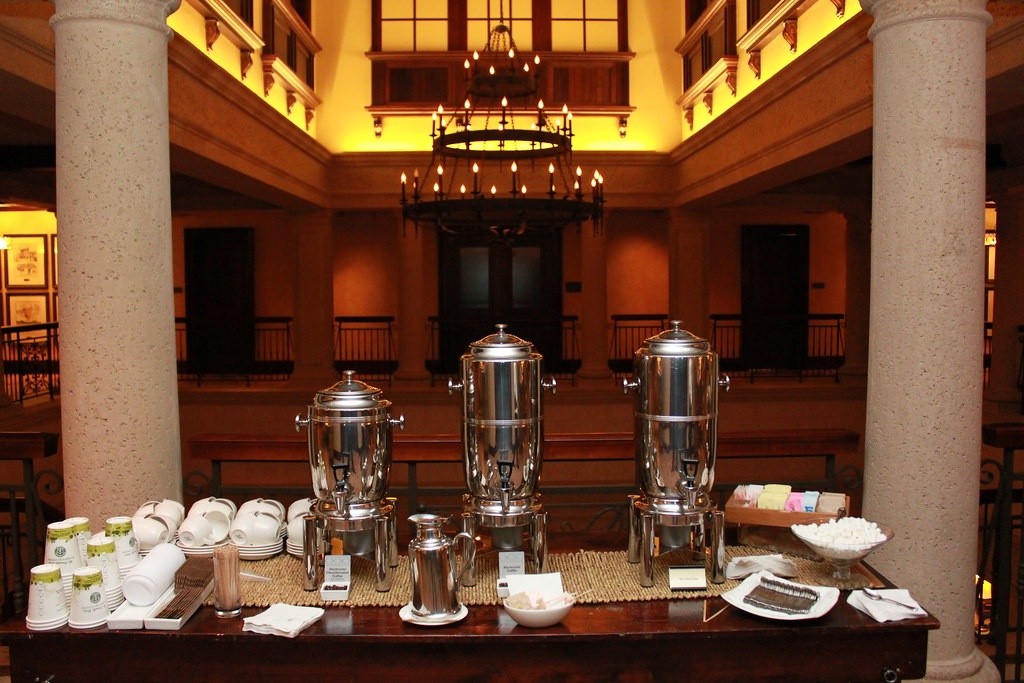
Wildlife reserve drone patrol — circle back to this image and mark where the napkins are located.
[725,552,798,580]
[846,589,928,623]
[242,603,325,640]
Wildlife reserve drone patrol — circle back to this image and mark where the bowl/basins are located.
[504,593,575,627]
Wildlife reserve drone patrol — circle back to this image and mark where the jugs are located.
[407,514,477,617]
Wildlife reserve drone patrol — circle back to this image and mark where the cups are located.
[210,550,241,619]
[180,498,316,546]
[26,496,184,631]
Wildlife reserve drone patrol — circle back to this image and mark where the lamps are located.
[401,0,607,248]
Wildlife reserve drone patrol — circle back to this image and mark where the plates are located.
[400,606,469,626]
[721,570,841,620]
[139,522,304,560]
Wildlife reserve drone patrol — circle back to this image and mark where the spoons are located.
[863,586,918,612]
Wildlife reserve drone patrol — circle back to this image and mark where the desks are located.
[0,529,939,683]
